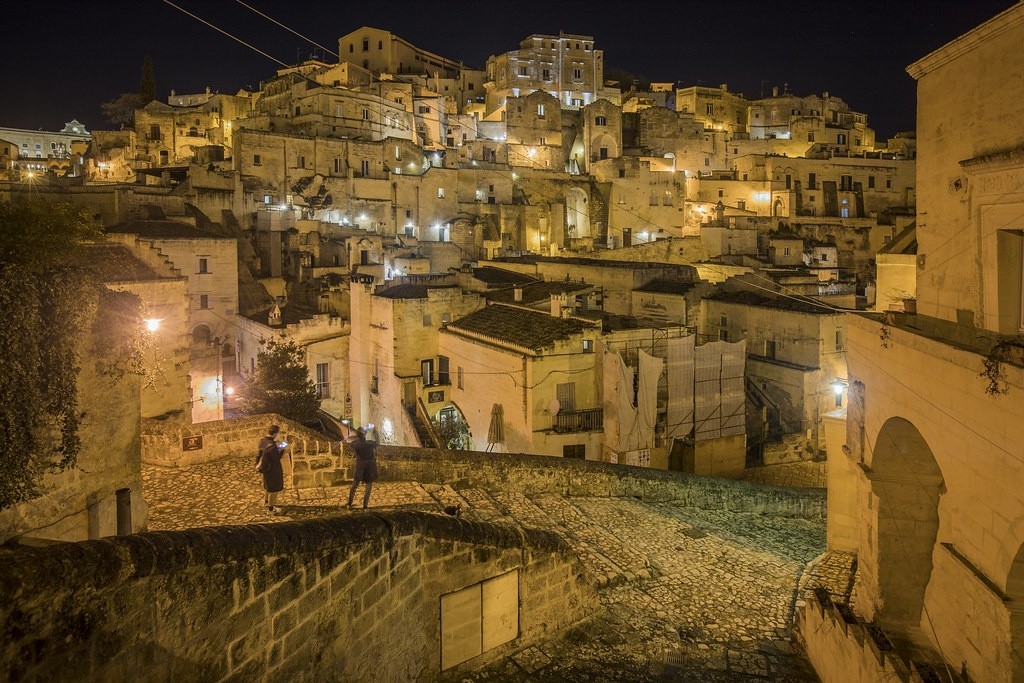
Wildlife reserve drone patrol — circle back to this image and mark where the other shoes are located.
[264,502,270,508]
[267,510,280,515]
[347,504,352,511]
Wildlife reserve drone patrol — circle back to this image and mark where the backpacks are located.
[256,444,274,473]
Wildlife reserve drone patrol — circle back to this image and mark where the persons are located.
[255,424,288,516]
[347,423,381,512]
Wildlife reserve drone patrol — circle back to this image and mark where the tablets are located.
[279,441,287,448]
[367,423,374,430]
